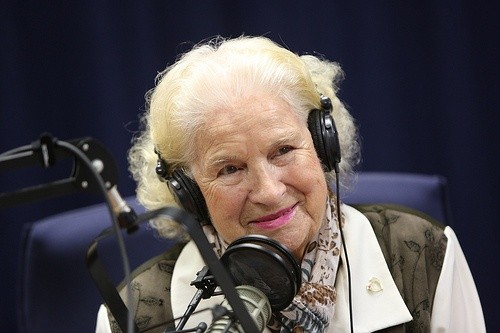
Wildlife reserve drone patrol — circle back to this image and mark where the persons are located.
[95,33,487,333]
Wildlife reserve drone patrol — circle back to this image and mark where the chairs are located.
[17,171,453,333]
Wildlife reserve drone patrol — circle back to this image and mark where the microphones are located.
[205,286,272,333]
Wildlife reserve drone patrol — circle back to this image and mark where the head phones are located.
[153,91,342,228]
